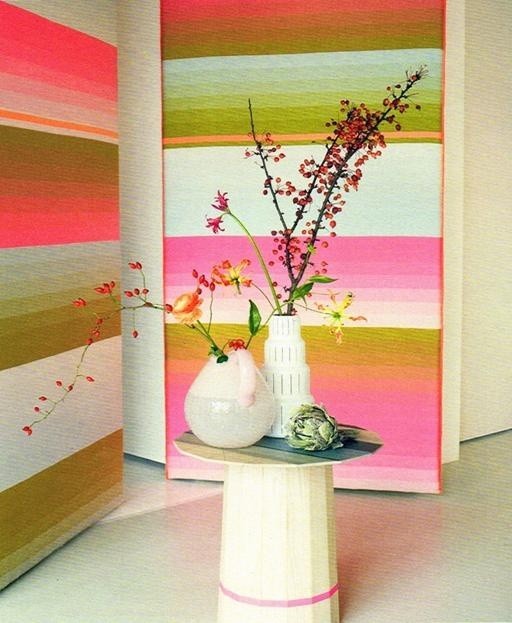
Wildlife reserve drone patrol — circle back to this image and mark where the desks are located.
[173,424,385,623]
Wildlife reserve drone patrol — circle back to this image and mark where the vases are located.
[183,348,277,450]
[258,316,311,438]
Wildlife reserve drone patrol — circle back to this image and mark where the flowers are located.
[22,258,338,435]
[283,404,360,452]
[205,65,430,347]
